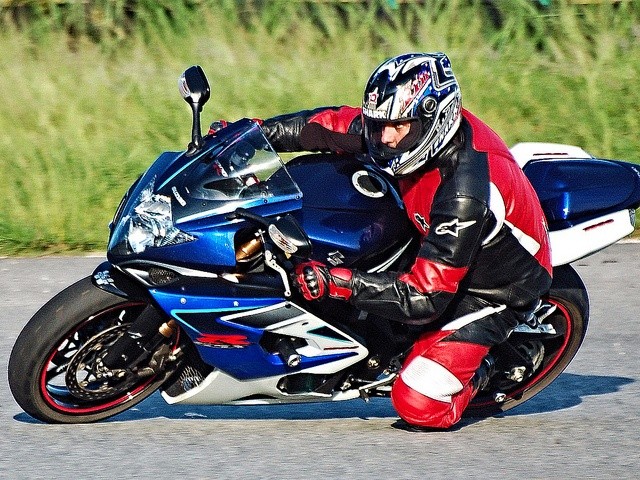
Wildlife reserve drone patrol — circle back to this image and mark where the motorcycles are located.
[8,67,640,424]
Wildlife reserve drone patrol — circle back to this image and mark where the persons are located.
[205,51,554,432]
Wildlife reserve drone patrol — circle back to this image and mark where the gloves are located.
[208,116,262,175]
[290,259,358,304]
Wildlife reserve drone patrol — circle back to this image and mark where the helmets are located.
[362,51,463,179]
